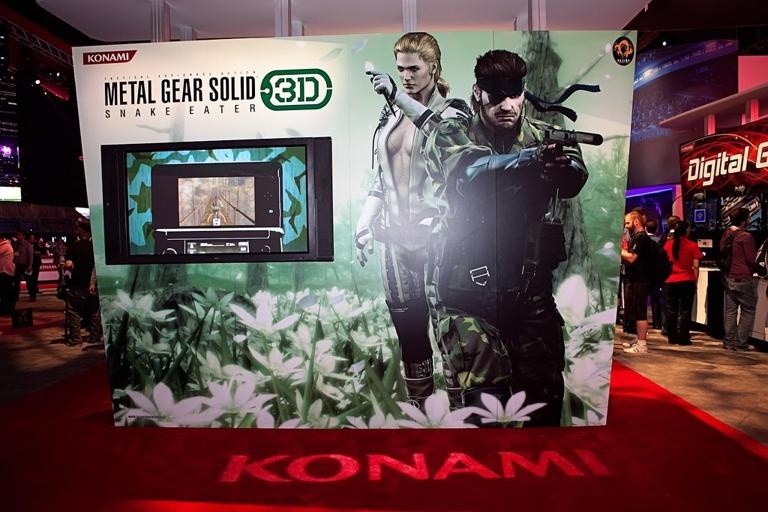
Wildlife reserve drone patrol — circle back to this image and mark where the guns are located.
[524,127,604,184]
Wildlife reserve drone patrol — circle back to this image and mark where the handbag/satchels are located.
[714,232,737,276]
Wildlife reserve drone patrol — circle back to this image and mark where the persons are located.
[355,32,474,413]
[0,223,102,347]
[720,208,757,351]
[621,206,702,353]
[424,49,590,427]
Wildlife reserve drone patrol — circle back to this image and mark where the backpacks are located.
[751,238,768,281]
[639,237,675,287]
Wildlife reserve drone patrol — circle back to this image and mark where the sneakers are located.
[622,345,650,355]
[734,343,757,353]
[65,335,83,348]
[722,341,736,350]
[667,334,693,347]
[622,341,639,348]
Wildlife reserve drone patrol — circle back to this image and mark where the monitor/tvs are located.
[100,137,334,264]
[717,193,764,231]
